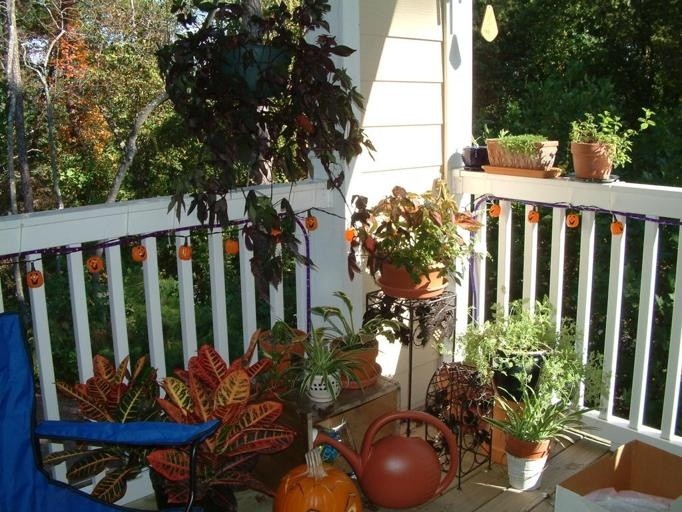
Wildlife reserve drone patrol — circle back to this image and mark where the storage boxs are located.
[554,431,682,512]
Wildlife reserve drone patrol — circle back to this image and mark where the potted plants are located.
[312,292,402,389]
[434,290,615,401]
[483,133,562,178]
[348,180,494,300]
[260,319,307,367]
[38,327,298,511]
[284,328,376,407]
[568,106,659,178]
[481,385,602,490]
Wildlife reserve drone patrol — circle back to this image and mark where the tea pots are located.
[312,408,461,510]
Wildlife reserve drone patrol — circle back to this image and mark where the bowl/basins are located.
[461,146,489,166]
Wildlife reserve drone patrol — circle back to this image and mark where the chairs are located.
[2,310,221,511]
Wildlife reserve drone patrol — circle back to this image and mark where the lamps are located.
[480,0,498,45]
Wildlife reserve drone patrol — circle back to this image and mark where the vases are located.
[460,144,491,173]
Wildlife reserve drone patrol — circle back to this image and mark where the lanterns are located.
[26,270,43,287]
[345,227,357,241]
[611,221,624,234]
[179,245,192,260]
[528,210,539,223]
[224,238,239,255]
[305,216,318,230]
[489,204,500,218]
[131,245,147,262]
[271,228,282,242]
[566,214,579,228]
[87,256,103,273]
